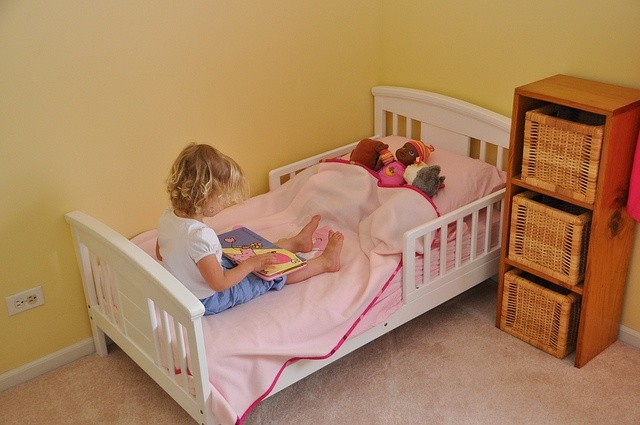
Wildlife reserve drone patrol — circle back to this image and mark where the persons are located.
[375,139,434,185]
[156,144,344,317]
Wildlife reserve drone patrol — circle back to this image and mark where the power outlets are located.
[4,285,46,317]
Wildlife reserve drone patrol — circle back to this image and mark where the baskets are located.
[507,189,592,287]
[499,267,581,359]
[521,103,604,206]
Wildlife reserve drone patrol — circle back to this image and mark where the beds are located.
[61,81,513,425]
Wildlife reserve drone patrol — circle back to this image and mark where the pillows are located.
[346,133,504,215]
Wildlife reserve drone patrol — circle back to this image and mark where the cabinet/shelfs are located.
[492,73,639,369]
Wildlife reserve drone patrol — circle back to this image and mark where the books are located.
[217,226,309,282]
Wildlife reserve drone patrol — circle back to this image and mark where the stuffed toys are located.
[349,138,388,170]
[403,161,446,198]
